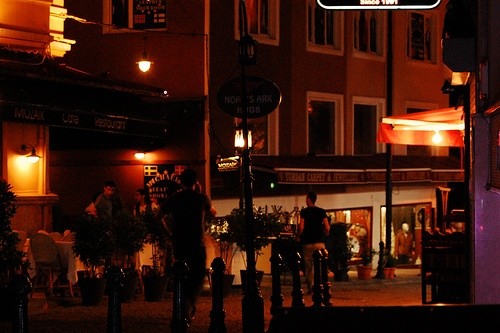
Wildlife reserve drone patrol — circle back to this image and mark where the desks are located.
[26,238,93,298]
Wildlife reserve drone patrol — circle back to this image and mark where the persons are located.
[133,186,160,217]
[84,181,122,217]
[297,192,331,295]
[412,207,426,264]
[395,223,413,264]
[154,169,217,327]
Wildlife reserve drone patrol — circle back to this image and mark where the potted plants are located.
[72,207,293,306]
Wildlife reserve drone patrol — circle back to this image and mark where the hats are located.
[179,169,199,179]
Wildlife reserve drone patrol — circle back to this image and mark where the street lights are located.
[238,0,265,330]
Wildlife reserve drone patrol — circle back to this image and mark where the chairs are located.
[30,229,68,299]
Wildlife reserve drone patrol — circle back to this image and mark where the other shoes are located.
[306,287,314,295]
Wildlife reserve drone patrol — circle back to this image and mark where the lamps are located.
[21,144,40,163]
[135,49,154,73]
